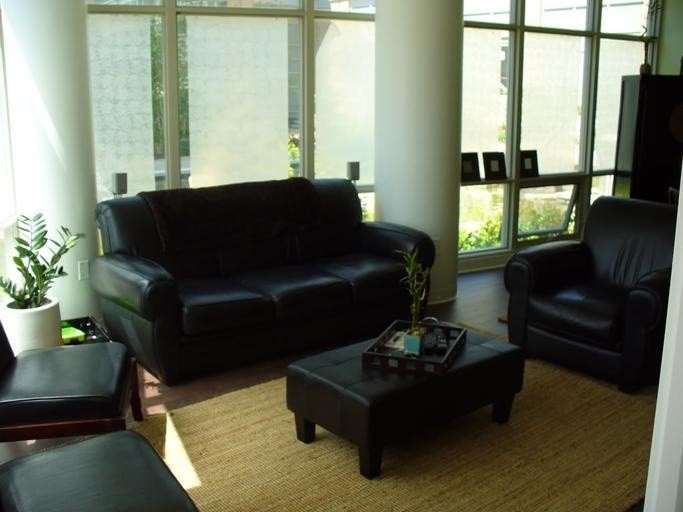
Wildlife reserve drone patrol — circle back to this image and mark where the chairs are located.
[0,319,152,454]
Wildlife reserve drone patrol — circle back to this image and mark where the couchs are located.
[92,175,438,388]
[0,426,200,511]
[501,193,677,395]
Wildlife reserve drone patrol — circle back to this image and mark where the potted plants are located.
[0,211,92,359]
[394,241,433,358]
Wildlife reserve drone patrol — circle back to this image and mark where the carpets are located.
[126,337,659,511]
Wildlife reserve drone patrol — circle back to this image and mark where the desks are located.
[284,313,528,481]
[55,314,112,347]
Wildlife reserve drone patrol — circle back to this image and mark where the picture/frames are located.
[460,150,481,182]
[482,151,508,180]
[520,150,539,178]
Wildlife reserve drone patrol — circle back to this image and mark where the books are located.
[59,327,85,345]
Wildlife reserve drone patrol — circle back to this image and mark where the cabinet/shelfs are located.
[610,72,683,204]
